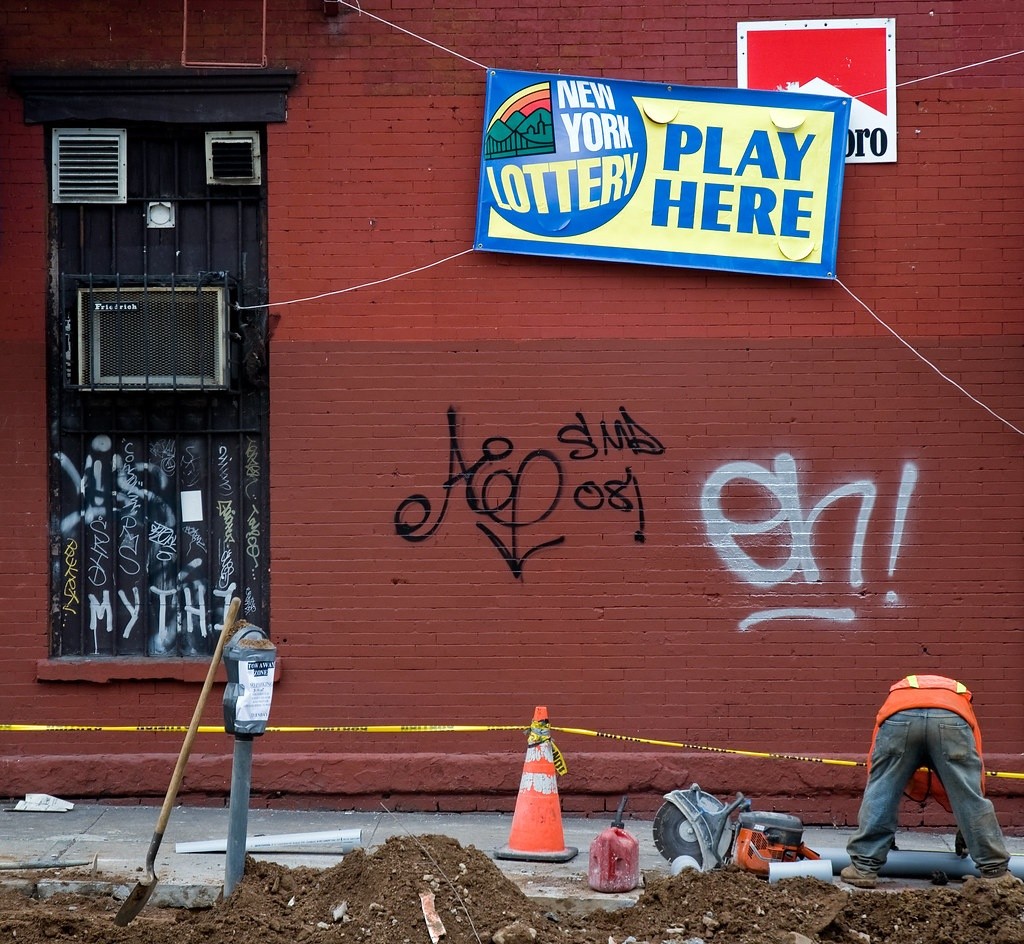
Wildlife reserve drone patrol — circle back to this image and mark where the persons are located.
[841,675,1024,889]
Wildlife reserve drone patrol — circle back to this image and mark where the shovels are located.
[111,594,245,931]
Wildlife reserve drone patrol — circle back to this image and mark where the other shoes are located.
[841,863,879,888]
[982,869,1023,886]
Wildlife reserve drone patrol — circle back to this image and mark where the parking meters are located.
[222,625,277,902]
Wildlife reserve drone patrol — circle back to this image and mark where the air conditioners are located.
[77,285,232,393]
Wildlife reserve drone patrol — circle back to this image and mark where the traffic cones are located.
[494,706,580,864]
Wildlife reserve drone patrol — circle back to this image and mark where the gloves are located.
[955,829,969,861]
[890,837,899,851]
